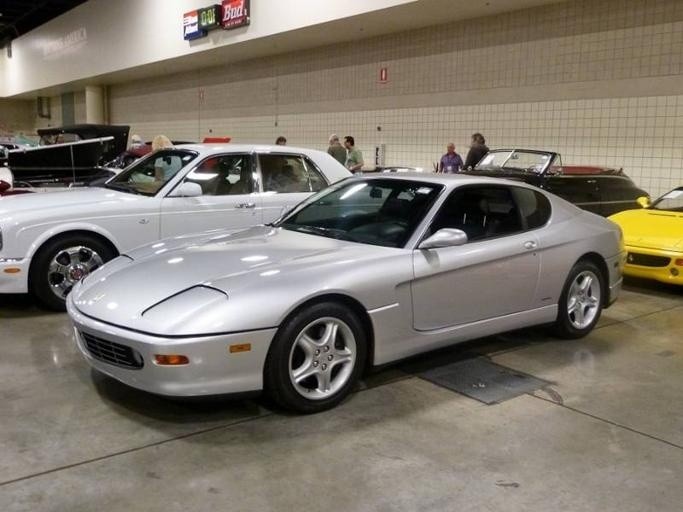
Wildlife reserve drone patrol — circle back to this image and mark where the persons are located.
[274,135,287,147]
[438,142,463,173]
[461,133,488,169]
[342,135,363,173]
[150,135,184,182]
[327,133,346,165]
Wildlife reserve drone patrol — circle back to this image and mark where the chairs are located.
[375,198,499,244]
[206,164,295,196]
